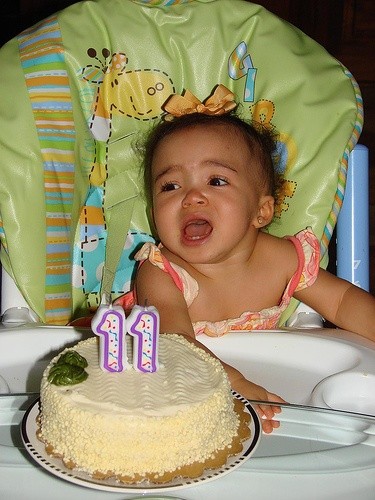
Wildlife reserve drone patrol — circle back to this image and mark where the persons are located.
[67,110,375,433]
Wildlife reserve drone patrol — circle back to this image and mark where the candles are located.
[90,292,129,373]
[124,297,159,372]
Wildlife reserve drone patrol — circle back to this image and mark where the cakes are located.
[35,330,252,485]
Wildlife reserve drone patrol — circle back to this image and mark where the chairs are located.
[0,0,375,499]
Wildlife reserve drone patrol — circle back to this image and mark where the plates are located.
[20,388,261,493]
